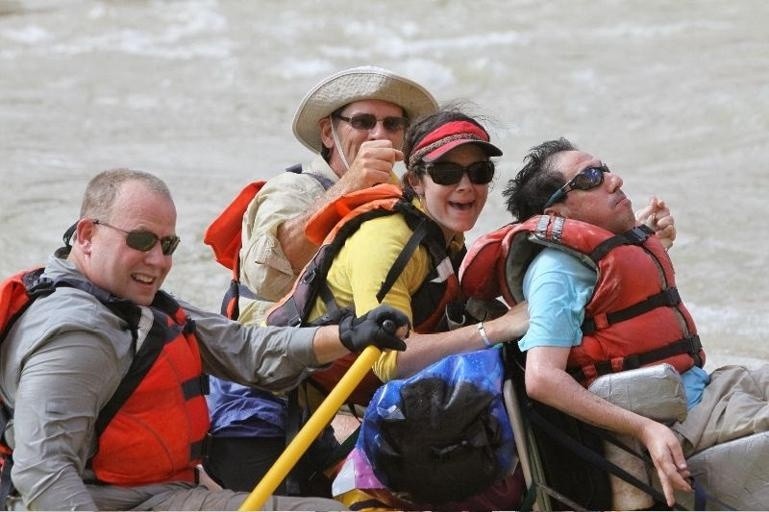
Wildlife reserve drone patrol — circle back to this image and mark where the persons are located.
[0,167,412,512]
[264,96,530,406]
[203,68,441,493]
[502,133,769,512]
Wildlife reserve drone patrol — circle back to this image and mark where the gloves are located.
[338,305,411,354]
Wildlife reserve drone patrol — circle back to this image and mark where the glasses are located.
[421,160,496,186]
[542,162,610,210]
[91,217,180,255]
[335,112,408,134]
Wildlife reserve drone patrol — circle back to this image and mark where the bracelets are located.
[478,322,491,346]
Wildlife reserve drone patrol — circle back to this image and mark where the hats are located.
[406,120,503,166]
[292,65,441,153]
